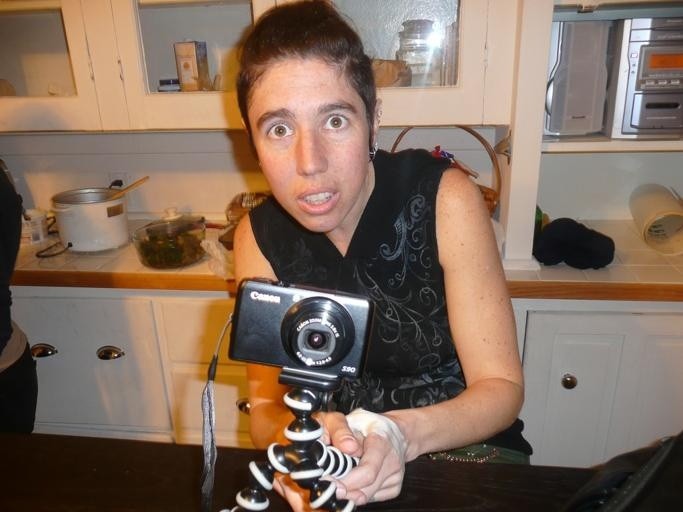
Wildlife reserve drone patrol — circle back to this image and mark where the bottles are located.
[397,20,439,86]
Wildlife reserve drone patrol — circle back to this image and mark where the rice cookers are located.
[50,180,129,254]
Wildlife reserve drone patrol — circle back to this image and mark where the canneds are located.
[157,78,181,92]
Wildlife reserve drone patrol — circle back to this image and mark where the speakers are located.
[543,22,615,135]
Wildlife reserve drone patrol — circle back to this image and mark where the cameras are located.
[229,280,377,380]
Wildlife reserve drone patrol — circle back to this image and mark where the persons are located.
[232,0,533,512]
[0,162,38,433]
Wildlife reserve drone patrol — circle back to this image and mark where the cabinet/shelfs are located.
[0,0,103,133]
[110,0,521,131]
[9,286,254,449]
[510,299,682,469]
[542,1,683,152]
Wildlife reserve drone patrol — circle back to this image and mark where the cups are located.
[18,209,48,248]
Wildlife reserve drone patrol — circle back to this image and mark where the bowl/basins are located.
[131,218,208,269]
[369,59,405,87]
